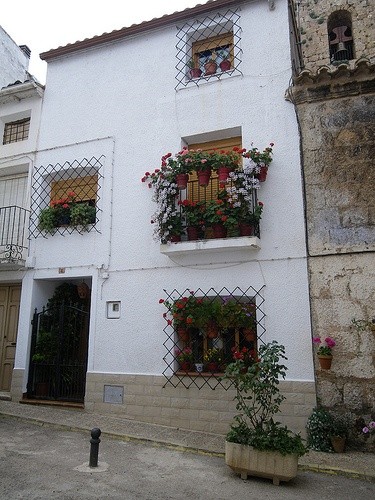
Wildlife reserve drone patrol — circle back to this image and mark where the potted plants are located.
[304,405,354,454]
[204,58,217,74]
[216,340,309,486]
[219,49,231,72]
[187,61,202,79]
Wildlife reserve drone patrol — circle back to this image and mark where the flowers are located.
[159,290,262,373]
[142,142,274,245]
[38,192,95,234]
[313,337,335,356]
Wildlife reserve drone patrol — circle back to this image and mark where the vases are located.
[186,227,198,241]
[171,235,181,242]
[195,363,203,372]
[178,325,189,341]
[216,166,231,182]
[180,361,190,370]
[176,173,189,190]
[208,323,218,338]
[318,355,333,370]
[258,167,268,182]
[212,224,226,237]
[196,168,212,187]
[239,224,253,236]
